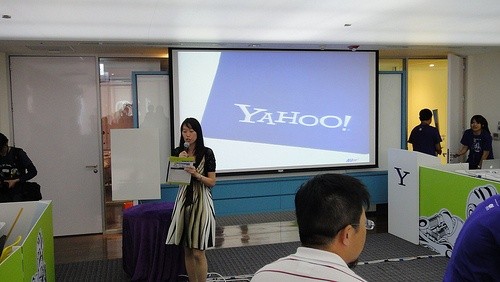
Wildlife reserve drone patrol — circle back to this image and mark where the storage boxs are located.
[0,200,54,282]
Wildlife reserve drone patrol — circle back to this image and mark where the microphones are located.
[184,141,190,153]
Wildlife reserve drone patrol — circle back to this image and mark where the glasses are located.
[343,220,375,230]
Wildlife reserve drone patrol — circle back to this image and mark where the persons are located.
[0,132,42,203]
[450,114,494,169]
[248,173,377,282]
[407,108,444,157]
[440,194,500,282]
[166,118,217,281]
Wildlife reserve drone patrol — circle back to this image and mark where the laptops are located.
[481,159,500,169]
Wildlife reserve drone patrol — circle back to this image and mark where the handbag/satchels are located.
[9,176,42,201]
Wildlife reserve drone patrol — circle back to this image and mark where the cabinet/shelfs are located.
[161,169,388,214]
[387,149,500,259]
